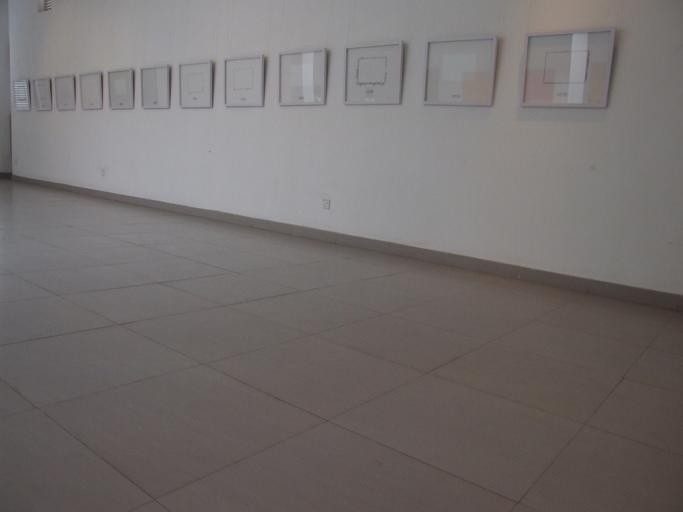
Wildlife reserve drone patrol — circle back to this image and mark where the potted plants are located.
[107,70,134,109]
[54,75,76,110]
[277,48,327,107]
[179,62,213,108]
[224,55,265,108]
[79,72,103,110]
[33,79,52,111]
[12,80,30,112]
[422,37,498,107]
[520,27,615,109]
[140,66,170,109]
[344,40,404,106]
